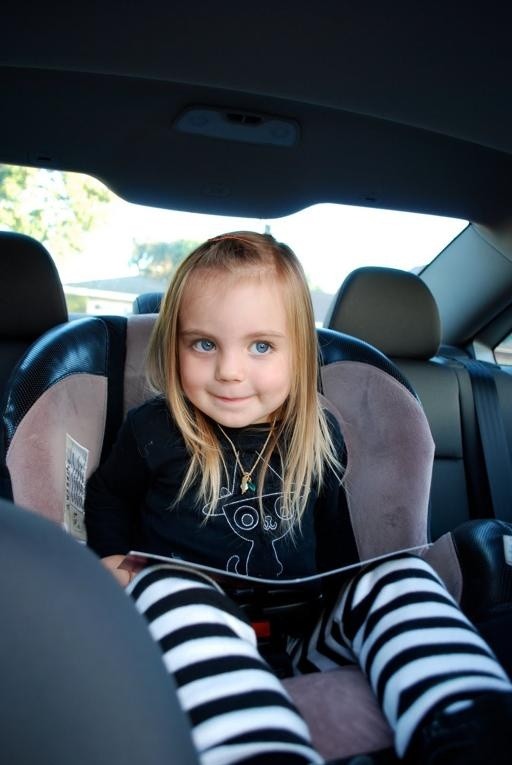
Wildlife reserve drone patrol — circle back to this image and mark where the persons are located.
[84,233,512,764]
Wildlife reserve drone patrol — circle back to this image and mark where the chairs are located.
[1,229,509,764]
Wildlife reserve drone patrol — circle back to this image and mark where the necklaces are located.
[218,404,281,495]
